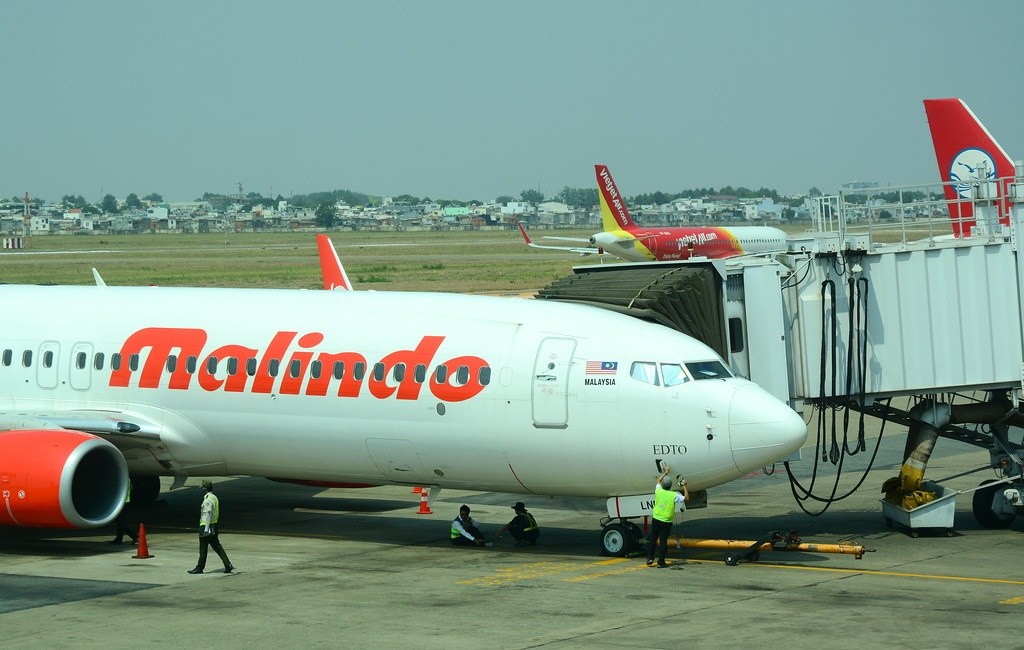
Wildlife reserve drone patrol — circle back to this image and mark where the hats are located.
[199,480,213,489]
[511,502,529,512]
[661,476,672,489]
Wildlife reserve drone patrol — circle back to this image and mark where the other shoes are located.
[528,541,538,545]
[223,564,234,573]
[513,541,528,547]
[188,565,203,574]
[646,559,653,565]
[658,563,668,567]
[484,542,494,547]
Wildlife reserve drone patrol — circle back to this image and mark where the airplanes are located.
[0,235,808,531]
[516,164,788,263]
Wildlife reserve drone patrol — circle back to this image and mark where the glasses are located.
[460,513,468,517]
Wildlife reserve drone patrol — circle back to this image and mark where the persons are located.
[110,477,139,545]
[647,468,689,568]
[451,505,496,550]
[496,502,539,547]
[188,480,234,574]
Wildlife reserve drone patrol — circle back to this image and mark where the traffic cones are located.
[132,522,155,559]
[411,486,424,493]
[416,487,433,515]
